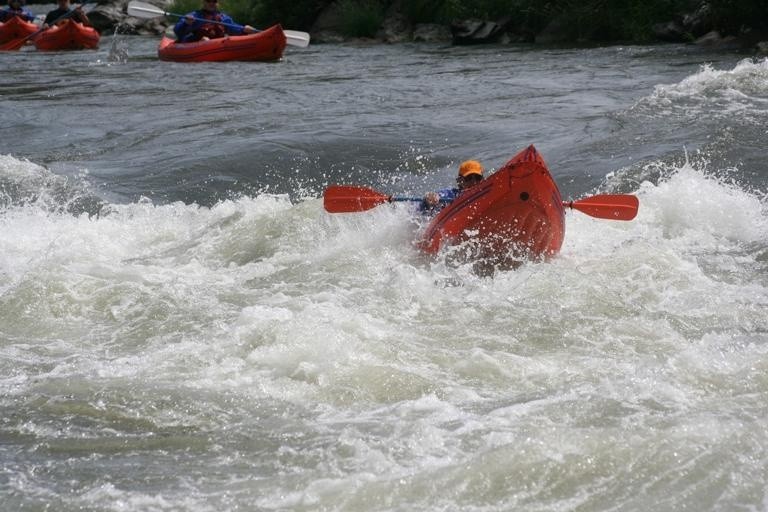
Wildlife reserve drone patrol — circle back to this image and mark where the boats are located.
[0,15,39,51]
[420,148,566,278]
[159,24,286,63]
[31,14,100,51]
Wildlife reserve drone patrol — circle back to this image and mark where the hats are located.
[458,160,483,178]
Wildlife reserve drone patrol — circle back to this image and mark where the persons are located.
[41,1,90,31]
[0,1,35,25]
[173,1,254,42]
[414,159,484,217]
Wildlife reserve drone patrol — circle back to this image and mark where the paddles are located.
[324,185,638,220]
[1,0,91,50]
[127,0,310,48]
